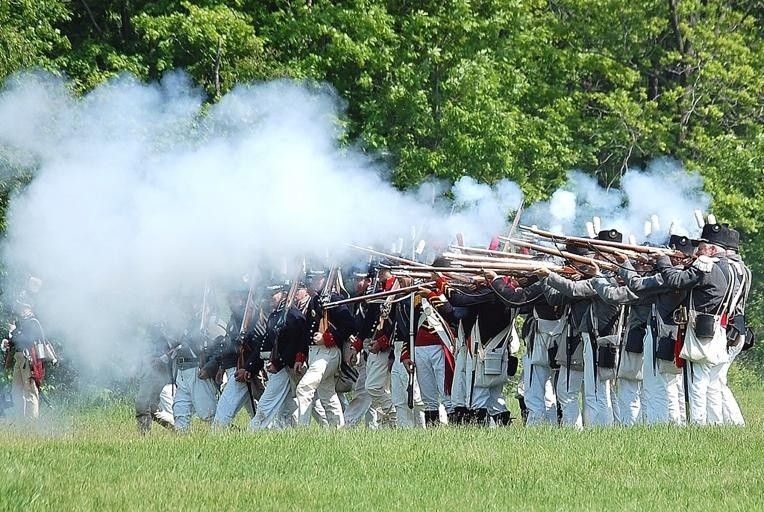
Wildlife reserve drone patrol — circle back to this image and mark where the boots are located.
[424,406,512,428]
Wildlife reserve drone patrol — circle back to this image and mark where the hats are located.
[599,229,622,243]
[666,235,694,255]
[727,229,740,251]
[264,252,402,295]
[431,255,450,267]
[691,223,730,250]
[566,236,592,256]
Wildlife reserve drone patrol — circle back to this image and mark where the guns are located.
[269,261,302,362]
[235,275,254,369]
[196,277,209,368]
[318,198,699,336]
[5,312,13,369]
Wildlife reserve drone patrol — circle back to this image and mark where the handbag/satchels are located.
[474,348,509,388]
[531,309,730,379]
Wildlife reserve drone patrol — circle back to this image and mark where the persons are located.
[128,222,753,435]
[0,296,46,427]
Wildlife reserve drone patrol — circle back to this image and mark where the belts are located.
[176,356,200,364]
